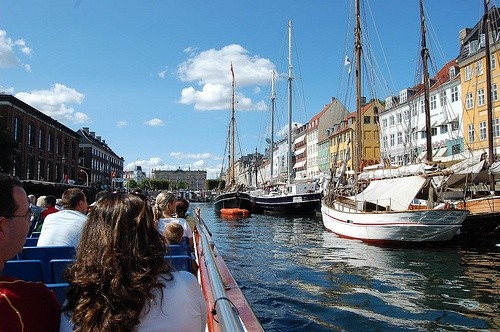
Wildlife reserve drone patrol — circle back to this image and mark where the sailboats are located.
[258,21,323,216]
[321,0,470,243]
[387,0,500,228]
[214,61,256,219]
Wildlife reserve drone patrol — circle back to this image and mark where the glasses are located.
[0,208,35,222]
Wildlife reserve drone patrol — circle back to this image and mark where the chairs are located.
[3,232,191,305]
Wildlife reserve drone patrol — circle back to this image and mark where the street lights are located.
[81,169,89,186]
